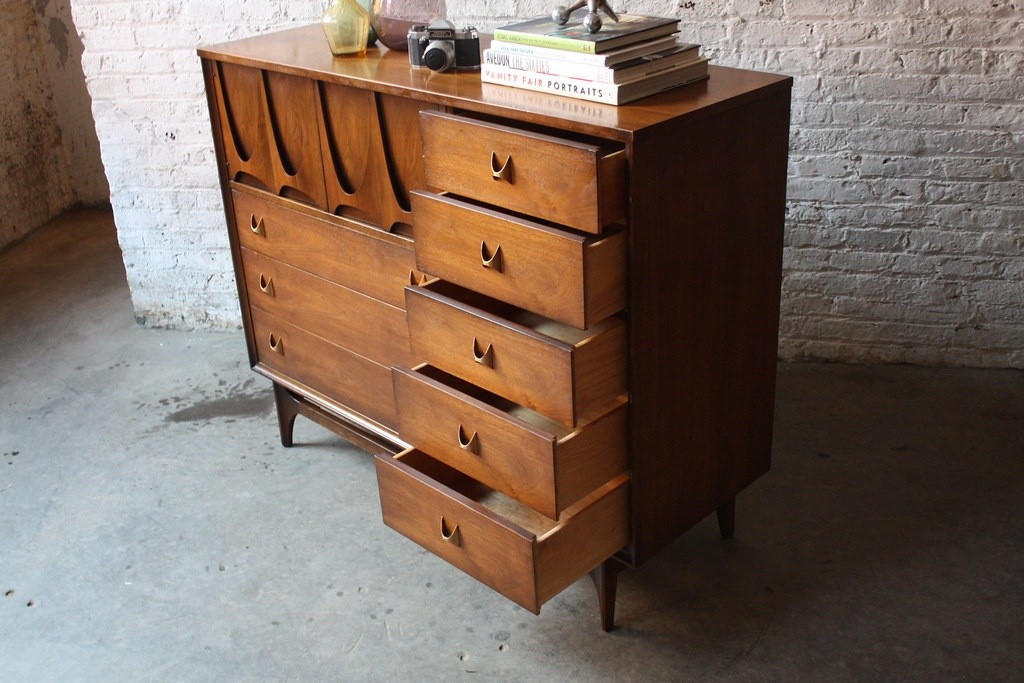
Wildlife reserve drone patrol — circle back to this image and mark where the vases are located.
[321,0,369,57]
[370,0,446,50]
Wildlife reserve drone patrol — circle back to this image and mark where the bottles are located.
[322,0,371,57]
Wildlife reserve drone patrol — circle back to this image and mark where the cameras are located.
[407,20,481,73]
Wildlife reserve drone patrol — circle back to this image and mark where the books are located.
[481,7,712,106]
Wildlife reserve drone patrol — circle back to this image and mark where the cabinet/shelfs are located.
[191,16,791,632]
